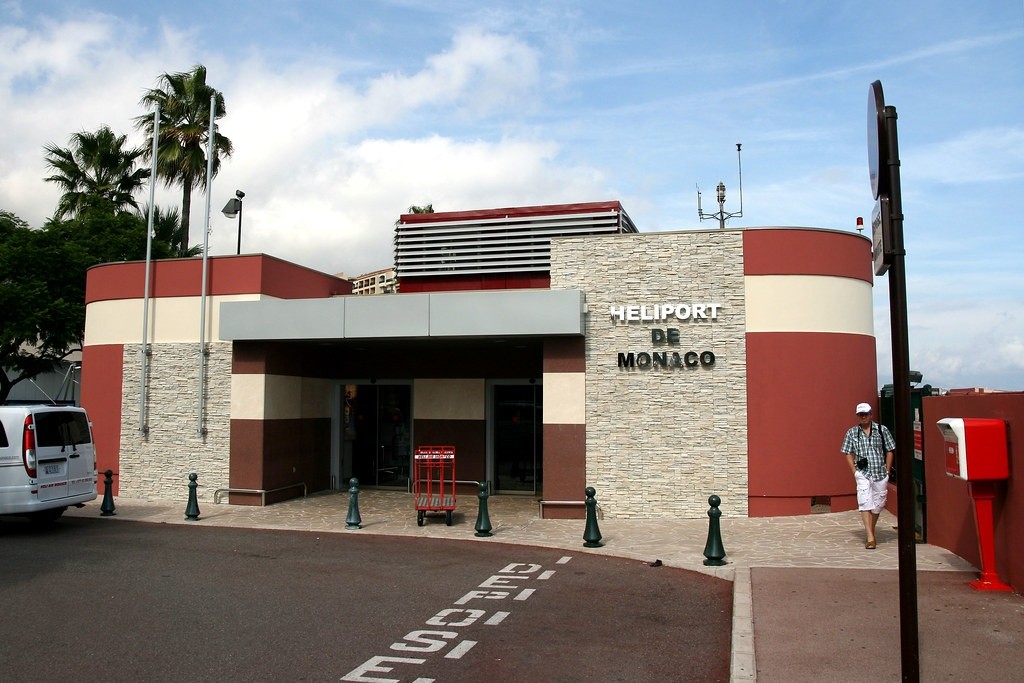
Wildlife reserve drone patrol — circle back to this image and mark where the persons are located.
[840,402,897,550]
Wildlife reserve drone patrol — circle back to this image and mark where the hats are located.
[856,403,872,414]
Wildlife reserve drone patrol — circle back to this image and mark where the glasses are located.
[857,412,870,416]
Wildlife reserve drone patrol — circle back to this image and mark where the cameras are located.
[857,457,868,470]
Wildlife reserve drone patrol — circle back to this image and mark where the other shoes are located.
[865,538,876,549]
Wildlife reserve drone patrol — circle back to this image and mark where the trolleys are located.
[414,446,456,526]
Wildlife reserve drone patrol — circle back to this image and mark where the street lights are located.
[221,189,245,255]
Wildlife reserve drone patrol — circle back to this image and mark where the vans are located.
[0,404,98,514]
